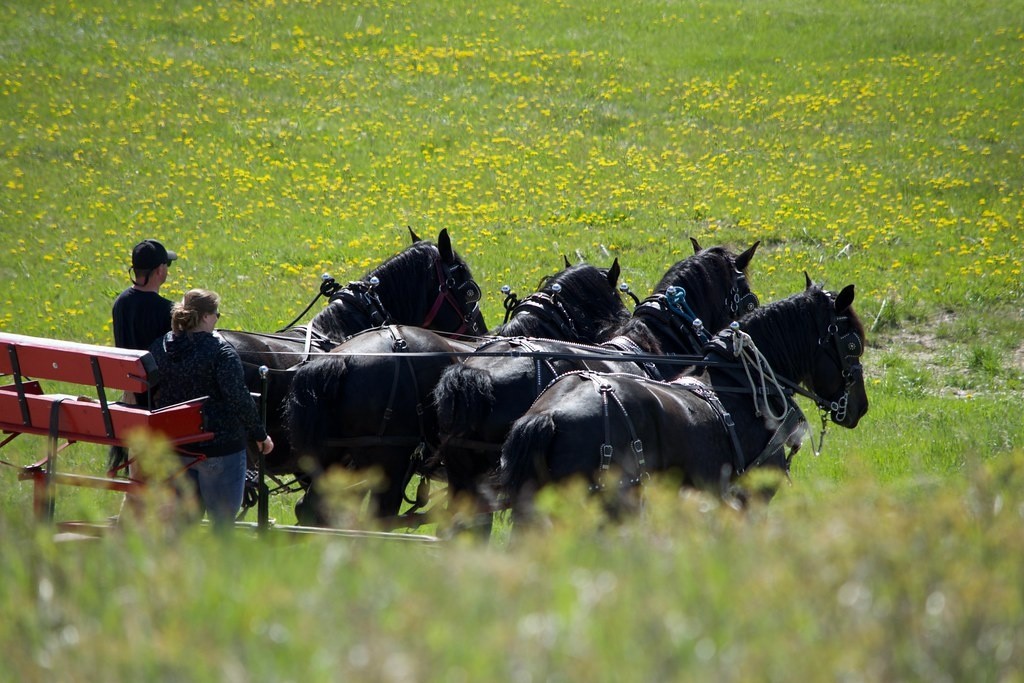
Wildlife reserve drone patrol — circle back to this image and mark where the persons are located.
[111,239,177,406]
[145,288,274,536]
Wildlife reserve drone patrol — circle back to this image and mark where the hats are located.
[132,240,178,269]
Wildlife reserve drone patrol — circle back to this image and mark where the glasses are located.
[209,312,220,319]
[163,260,172,267]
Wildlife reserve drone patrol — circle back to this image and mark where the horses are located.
[96,225,870,550]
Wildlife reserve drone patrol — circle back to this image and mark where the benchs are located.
[0,332,214,447]
